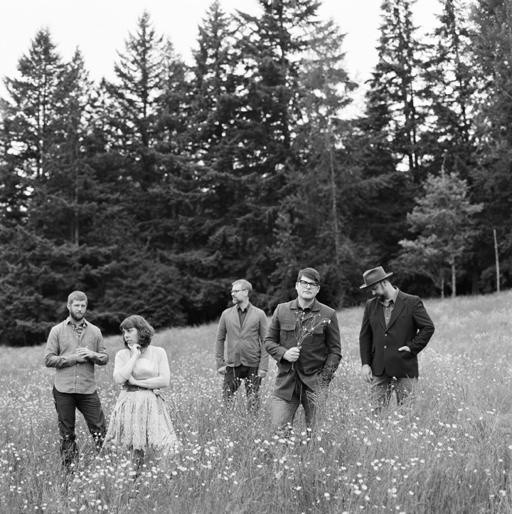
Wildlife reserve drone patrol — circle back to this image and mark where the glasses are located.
[231,289,245,293]
[298,280,319,286]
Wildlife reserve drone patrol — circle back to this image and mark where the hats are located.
[359,266,393,290]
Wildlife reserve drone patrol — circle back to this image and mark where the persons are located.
[215,279,269,412]
[359,265,434,414]
[263,267,341,451]
[100,315,170,474]
[45,290,109,470]
